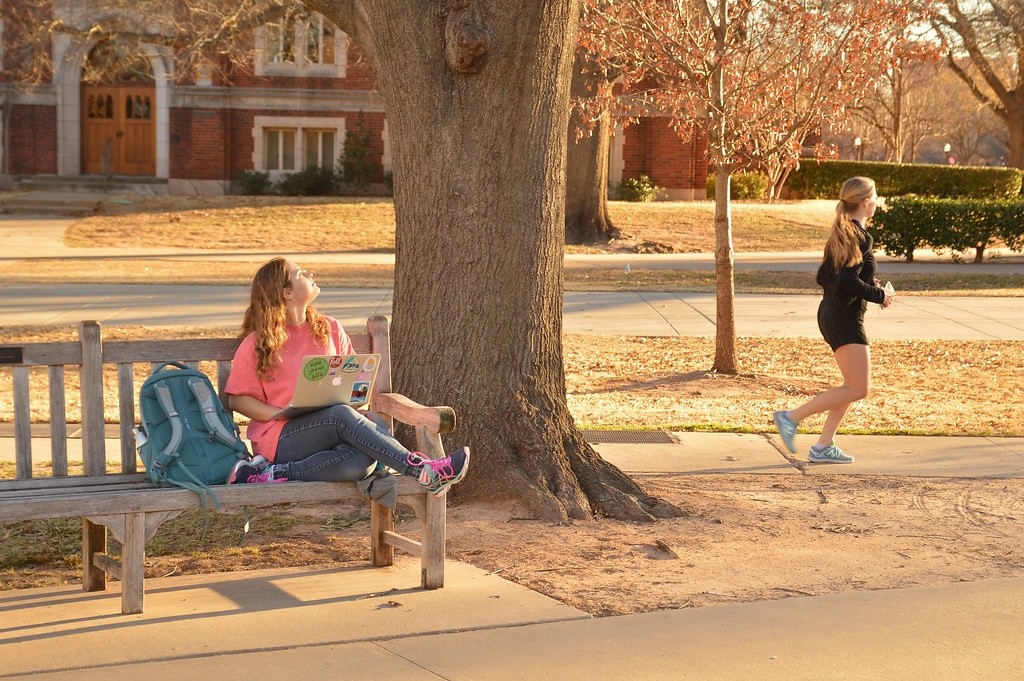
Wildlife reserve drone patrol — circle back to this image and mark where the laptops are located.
[270,354,381,421]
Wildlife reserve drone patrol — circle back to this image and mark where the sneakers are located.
[407,446,470,498]
[807,441,854,464]
[773,411,800,454]
[225,455,288,485]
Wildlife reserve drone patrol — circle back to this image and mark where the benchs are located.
[0,316,458,613]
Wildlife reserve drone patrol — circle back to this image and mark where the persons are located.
[772,176,895,464]
[225,256,470,498]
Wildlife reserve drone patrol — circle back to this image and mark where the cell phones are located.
[884,281,894,297]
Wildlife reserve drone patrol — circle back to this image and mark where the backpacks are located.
[131,361,253,487]
